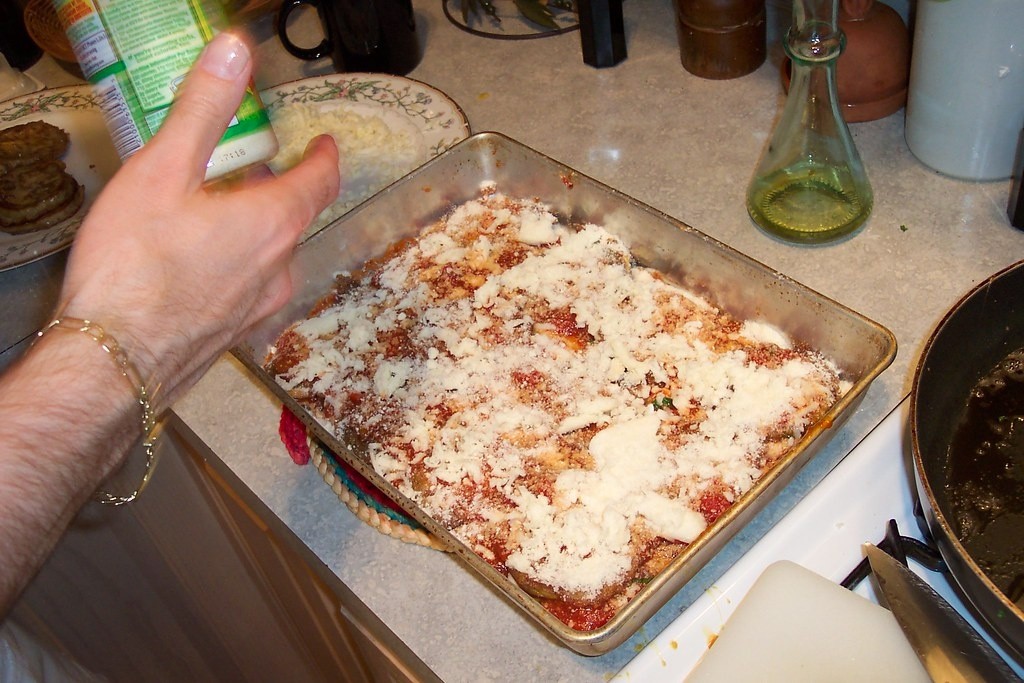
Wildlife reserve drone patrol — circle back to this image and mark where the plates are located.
[257,71,471,244]
[0,83,121,272]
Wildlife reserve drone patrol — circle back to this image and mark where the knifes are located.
[864,539,1024,683]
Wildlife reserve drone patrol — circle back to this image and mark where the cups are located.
[278,0,420,75]
[903,0,1024,183]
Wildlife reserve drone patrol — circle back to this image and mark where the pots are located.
[909,256,1024,666]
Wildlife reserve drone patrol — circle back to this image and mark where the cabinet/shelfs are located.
[156,416,425,683]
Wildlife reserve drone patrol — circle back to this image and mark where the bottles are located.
[50,0,279,187]
[747,1,874,245]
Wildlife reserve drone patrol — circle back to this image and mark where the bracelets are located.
[24,315,164,508]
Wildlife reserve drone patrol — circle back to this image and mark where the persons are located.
[0,31,341,628]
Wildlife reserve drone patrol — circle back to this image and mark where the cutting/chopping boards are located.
[688,560,935,683]
